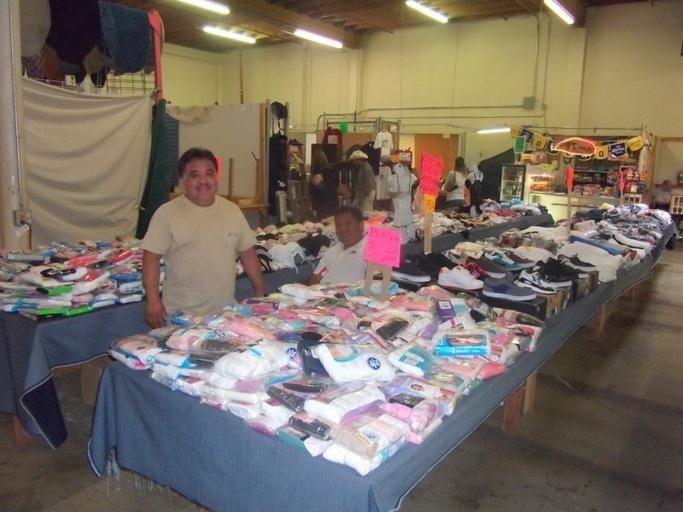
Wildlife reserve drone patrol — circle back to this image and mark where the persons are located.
[439,157,503,216]
[141,147,264,329]
[300,206,368,286]
[385,164,417,226]
[309,149,339,217]
[346,150,376,214]
[653,179,682,251]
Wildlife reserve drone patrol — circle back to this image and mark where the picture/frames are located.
[498,164,526,203]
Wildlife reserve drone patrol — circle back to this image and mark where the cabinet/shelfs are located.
[511,131,657,222]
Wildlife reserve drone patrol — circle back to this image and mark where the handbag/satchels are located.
[444,178,457,191]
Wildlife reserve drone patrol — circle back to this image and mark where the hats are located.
[347,150,368,161]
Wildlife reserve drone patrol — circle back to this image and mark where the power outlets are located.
[14,208,33,227]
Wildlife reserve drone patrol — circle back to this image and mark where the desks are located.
[0,196,678,512]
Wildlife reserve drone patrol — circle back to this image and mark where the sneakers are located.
[391,249,595,301]
